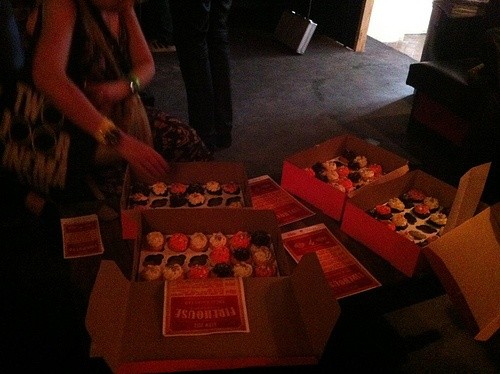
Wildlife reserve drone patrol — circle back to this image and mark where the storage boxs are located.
[340,168,500,342]
[280,134,409,223]
[83,208,340,374]
[273,7,317,55]
[120,162,254,258]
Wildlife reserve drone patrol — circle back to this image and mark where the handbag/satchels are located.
[0,0,100,205]
[98,92,154,163]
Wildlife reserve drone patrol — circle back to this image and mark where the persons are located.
[32,0,169,280]
[0,0,24,70]
[171,0,232,151]
[26,0,41,35]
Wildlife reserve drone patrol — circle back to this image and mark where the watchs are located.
[103,126,123,147]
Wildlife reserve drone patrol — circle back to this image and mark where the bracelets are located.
[129,71,140,93]
[93,118,114,144]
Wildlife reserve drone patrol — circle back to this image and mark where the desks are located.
[408,55,500,201]
[0,164,500,374]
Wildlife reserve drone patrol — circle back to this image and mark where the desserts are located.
[130,181,243,209]
[304,150,383,192]
[139,230,278,280]
[369,188,450,247]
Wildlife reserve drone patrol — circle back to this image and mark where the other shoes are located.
[192,127,232,152]
